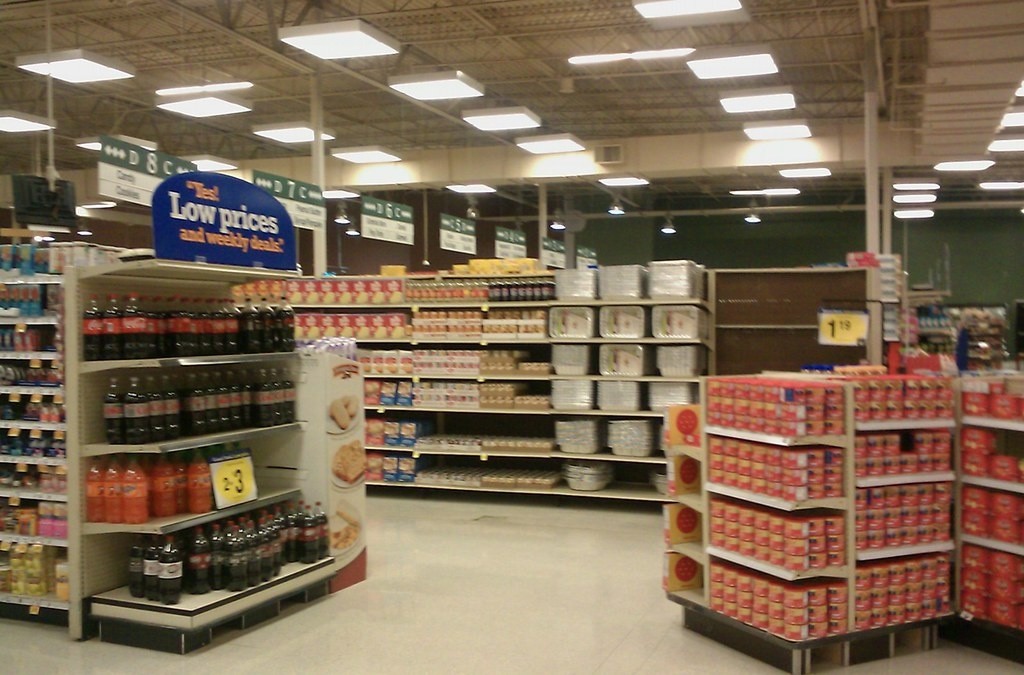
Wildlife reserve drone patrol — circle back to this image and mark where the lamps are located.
[515,133,585,154]
[465,196,480,219]
[607,194,625,214]
[334,199,350,224]
[386,39,486,99]
[75,134,158,150]
[550,207,567,229]
[686,46,781,79]
[0,110,57,133]
[719,85,796,113]
[330,145,404,163]
[277,0,401,60]
[155,92,252,117]
[179,154,238,172]
[659,215,677,233]
[16,50,135,85]
[461,106,543,131]
[744,119,813,140]
[745,197,761,222]
[251,121,335,144]
[345,214,360,236]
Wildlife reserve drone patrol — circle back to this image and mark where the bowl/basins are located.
[562,462,615,490]
[649,471,667,494]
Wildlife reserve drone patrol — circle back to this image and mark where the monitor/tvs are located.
[12,175,76,227]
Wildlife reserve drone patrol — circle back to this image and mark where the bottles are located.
[126,500,330,606]
[83,292,297,360]
[405,277,556,303]
[86,443,249,522]
[102,366,297,445]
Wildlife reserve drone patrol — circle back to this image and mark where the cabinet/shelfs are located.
[1,241,1024,674]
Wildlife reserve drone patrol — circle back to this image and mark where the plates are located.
[330,471,365,489]
[326,412,360,435]
[325,531,363,557]
